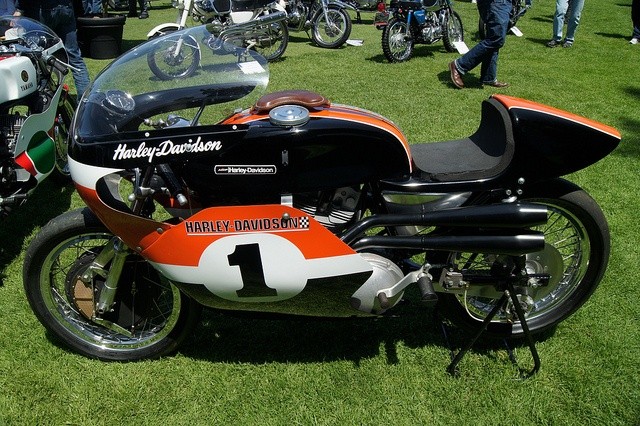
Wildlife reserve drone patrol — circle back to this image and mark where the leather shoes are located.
[449,61,465,89]
[482,81,508,87]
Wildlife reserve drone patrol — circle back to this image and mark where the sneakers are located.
[629,38,639,44]
[563,39,574,48]
[546,40,561,47]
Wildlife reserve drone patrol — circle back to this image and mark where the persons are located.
[9,0,89,101]
[81,0,102,15]
[544,0,586,47]
[448,0,513,89]
[124,0,149,20]
[1,0,19,37]
[629,0,640,43]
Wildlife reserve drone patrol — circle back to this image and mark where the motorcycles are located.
[381,0,465,63]
[145,0,291,79]
[20,21,624,375]
[285,0,360,48]
[0,12,77,226]
